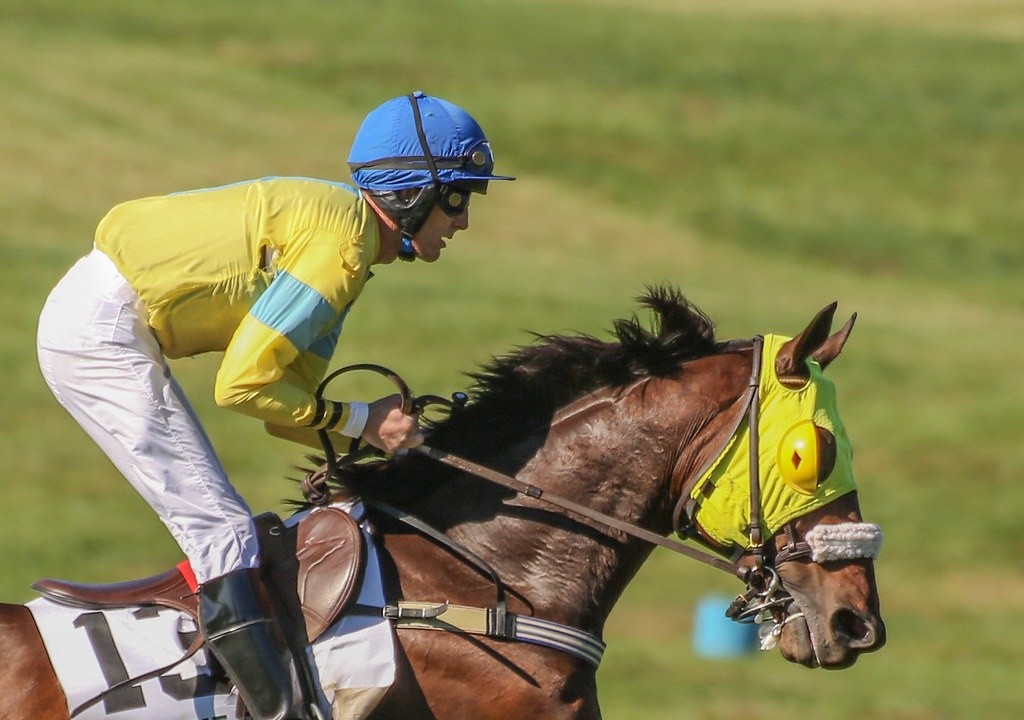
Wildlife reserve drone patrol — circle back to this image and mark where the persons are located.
[36,88,494,720]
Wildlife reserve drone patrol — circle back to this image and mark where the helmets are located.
[347,89,515,194]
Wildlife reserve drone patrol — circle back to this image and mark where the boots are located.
[198,567,322,720]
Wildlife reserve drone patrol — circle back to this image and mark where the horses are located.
[0,283,886,719]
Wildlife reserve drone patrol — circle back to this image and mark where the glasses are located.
[437,184,473,219]
